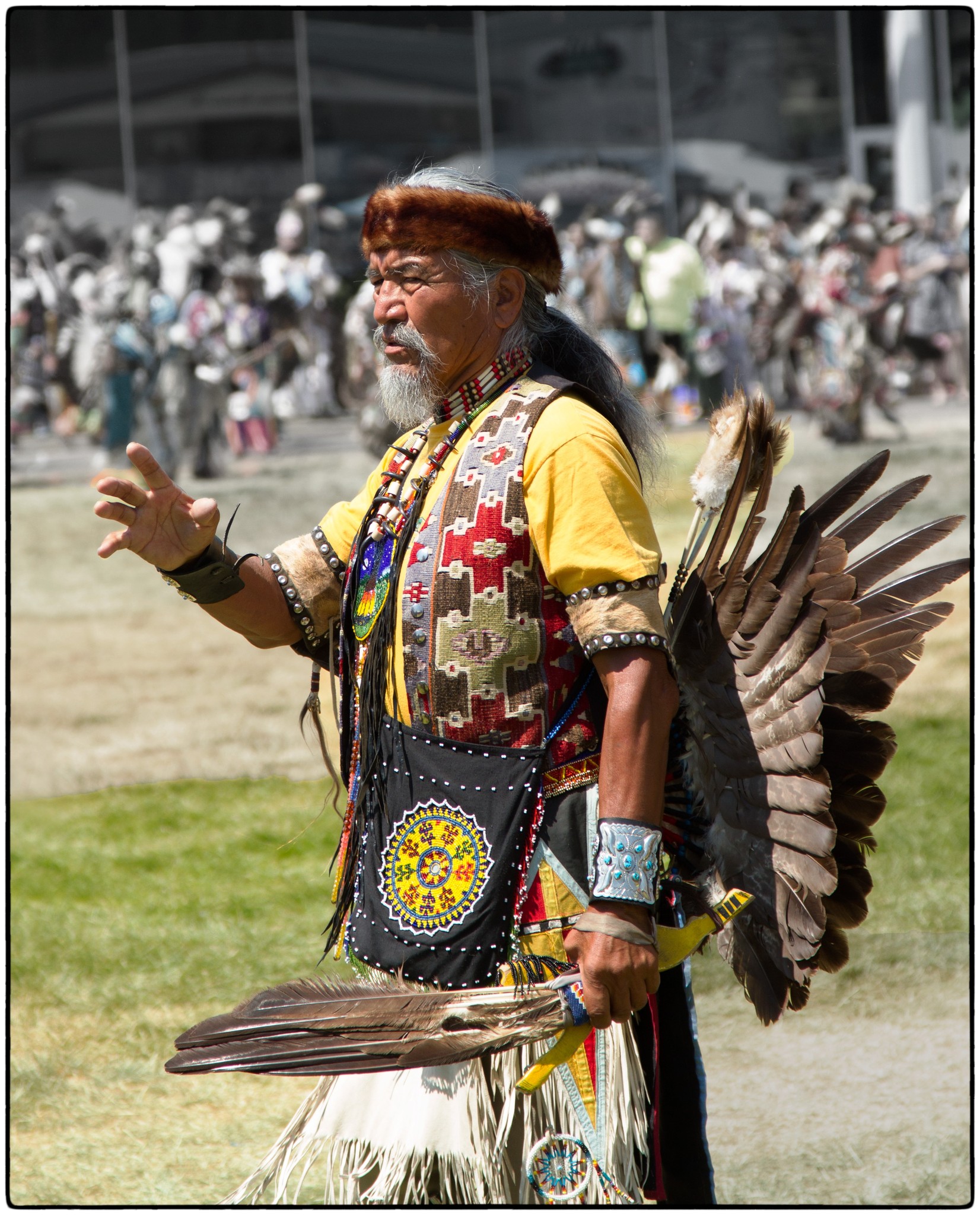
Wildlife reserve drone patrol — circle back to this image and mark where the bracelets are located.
[589,817,665,908]
[156,533,264,604]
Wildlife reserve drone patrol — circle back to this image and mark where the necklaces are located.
[336,343,532,538]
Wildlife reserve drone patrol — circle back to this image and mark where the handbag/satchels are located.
[343,707,549,992]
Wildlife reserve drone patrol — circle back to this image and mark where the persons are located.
[95,162,716,1215]
[10,182,968,488]
